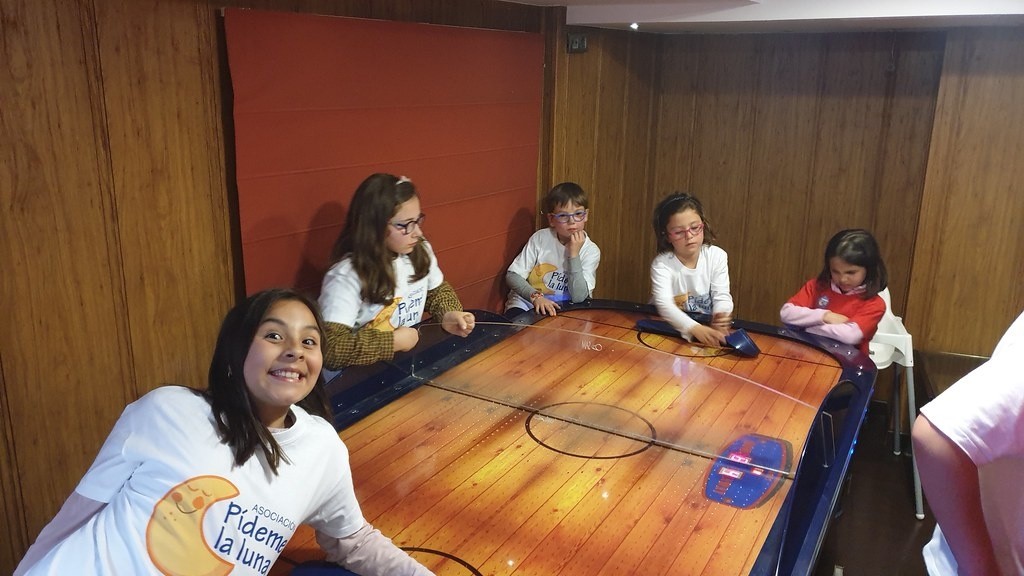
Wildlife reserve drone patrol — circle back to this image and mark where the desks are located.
[266,300,876,576]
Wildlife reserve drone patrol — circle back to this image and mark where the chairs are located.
[869,286,926,518]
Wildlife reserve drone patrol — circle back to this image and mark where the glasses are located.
[387,213,426,235]
[666,220,704,241]
[551,209,587,223]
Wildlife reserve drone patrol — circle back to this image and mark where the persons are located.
[650,189,735,349]
[777,227,889,518]
[9,287,437,576]
[908,308,1024,576]
[314,172,476,386]
[504,182,601,323]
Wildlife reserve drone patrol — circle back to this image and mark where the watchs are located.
[529,290,544,304]
